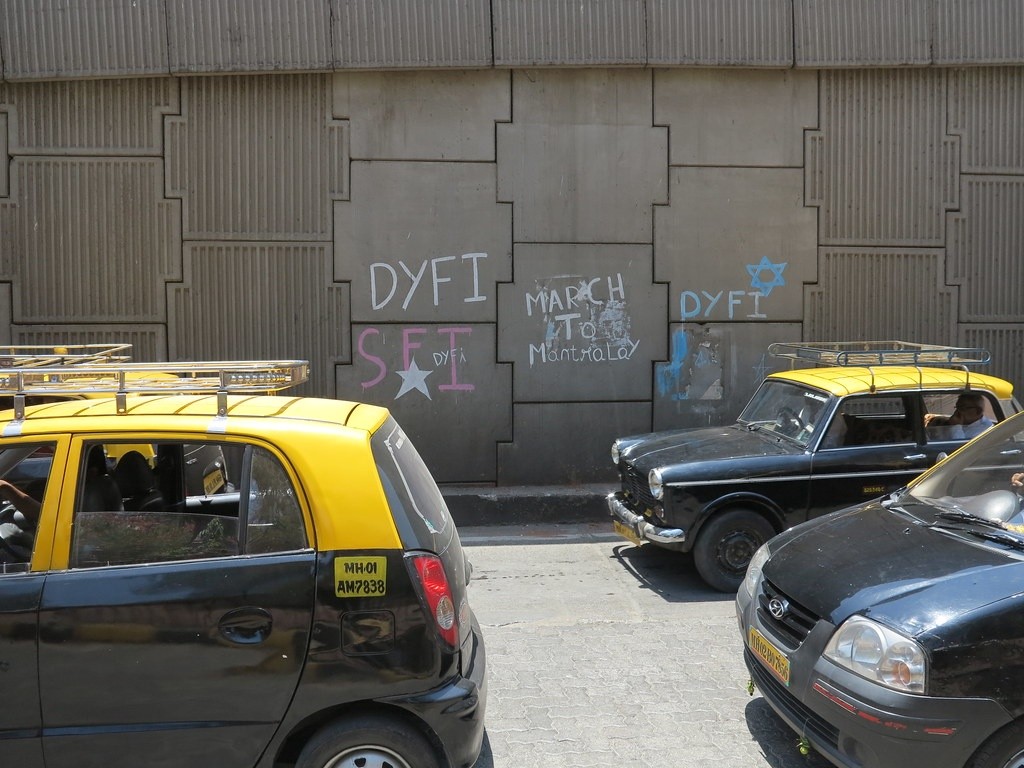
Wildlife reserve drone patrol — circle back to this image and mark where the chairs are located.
[113,447,168,550]
[76,460,126,569]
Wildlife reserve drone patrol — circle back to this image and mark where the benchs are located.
[841,412,914,446]
[923,412,953,429]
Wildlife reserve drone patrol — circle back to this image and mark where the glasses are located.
[954,404,978,412]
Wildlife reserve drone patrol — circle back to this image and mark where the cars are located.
[604,339,1024,594]
[727,407,1024,767]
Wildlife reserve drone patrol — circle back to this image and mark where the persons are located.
[924,393,992,441]
[0,479,41,527]
[1011,473,1024,487]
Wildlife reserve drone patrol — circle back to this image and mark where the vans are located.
[1,340,489,768]
